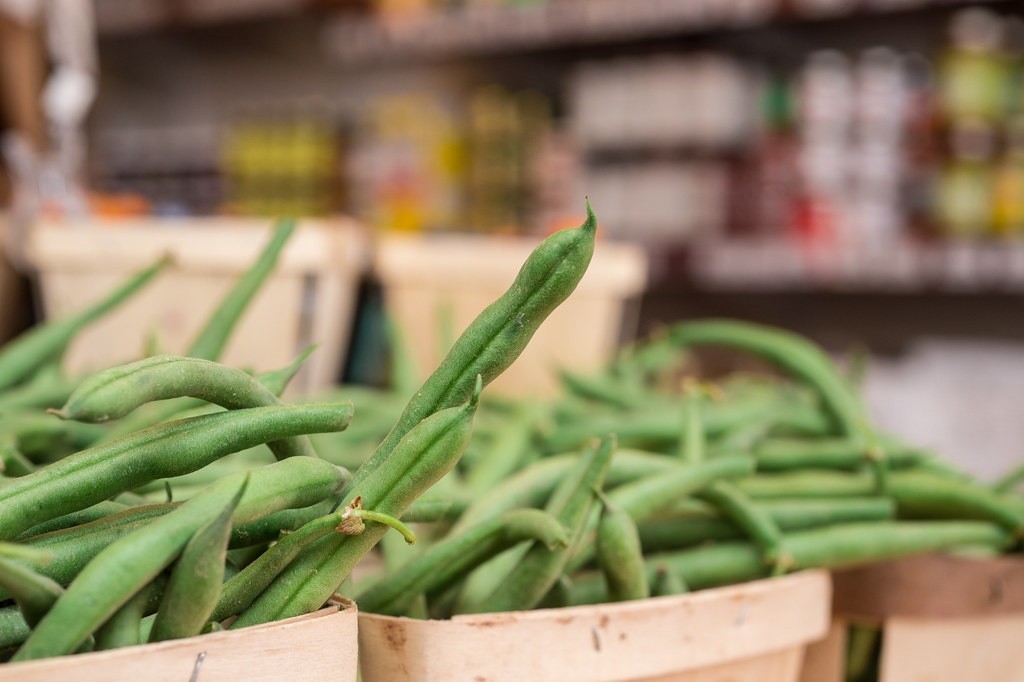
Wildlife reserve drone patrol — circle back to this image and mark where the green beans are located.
[0,197,1023,681]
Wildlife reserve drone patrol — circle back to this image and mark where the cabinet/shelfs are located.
[322,1,1023,296]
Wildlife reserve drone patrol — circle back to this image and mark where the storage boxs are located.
[372,231,649,395]
[0,557,1024,681]
[24,218,359,386]
